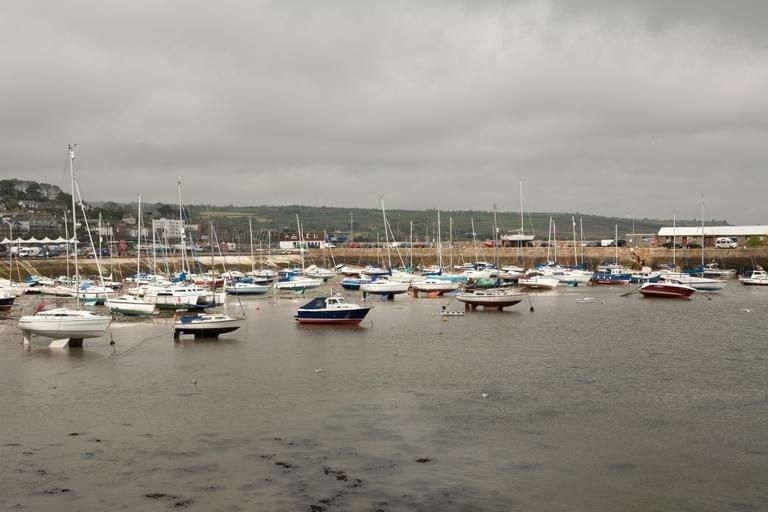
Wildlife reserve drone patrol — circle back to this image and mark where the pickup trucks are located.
[663,243,682,249]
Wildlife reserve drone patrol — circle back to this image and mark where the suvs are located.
[611,239,626,247]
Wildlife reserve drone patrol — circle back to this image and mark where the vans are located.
[325,243,337,248]
[714,237,738,249]
[18,247,41,258]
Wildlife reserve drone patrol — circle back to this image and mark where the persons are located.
[81,295,87,309]
[466,277,476,293]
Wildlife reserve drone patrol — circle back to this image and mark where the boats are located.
[738,254,768,285]
[170,312,240,341]
[637,279,697,297]
[453,287,526,312]
[292,296,374,326]
[16,143,112,349]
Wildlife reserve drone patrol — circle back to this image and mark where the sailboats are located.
[675,199,728,291]
[0,177,737,317]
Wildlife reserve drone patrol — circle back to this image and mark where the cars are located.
[686,241,702,249]
